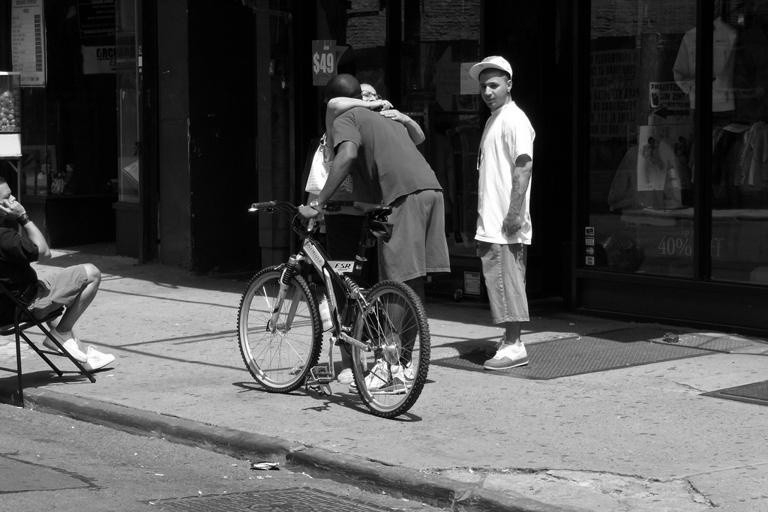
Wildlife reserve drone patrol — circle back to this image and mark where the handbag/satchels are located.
[305,133,352,194]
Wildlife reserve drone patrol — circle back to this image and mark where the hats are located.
[469,56,512,82]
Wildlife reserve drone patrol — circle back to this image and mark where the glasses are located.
[362,90,383,99]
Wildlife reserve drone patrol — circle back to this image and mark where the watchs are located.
[308,197,322,212]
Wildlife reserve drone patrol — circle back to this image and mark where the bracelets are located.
[17,212,30,228]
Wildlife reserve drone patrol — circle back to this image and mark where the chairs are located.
[0,298,97,408]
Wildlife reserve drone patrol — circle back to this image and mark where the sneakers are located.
[43,327,116,371]
[337,359,415,395]
[483,339,530,370]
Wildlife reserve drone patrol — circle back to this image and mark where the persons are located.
[295,73,455,398]
[304,83,426,385]
[0,175,115,374]
[468,54,536,372]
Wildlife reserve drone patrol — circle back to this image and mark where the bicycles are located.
[237,199,431,418]
